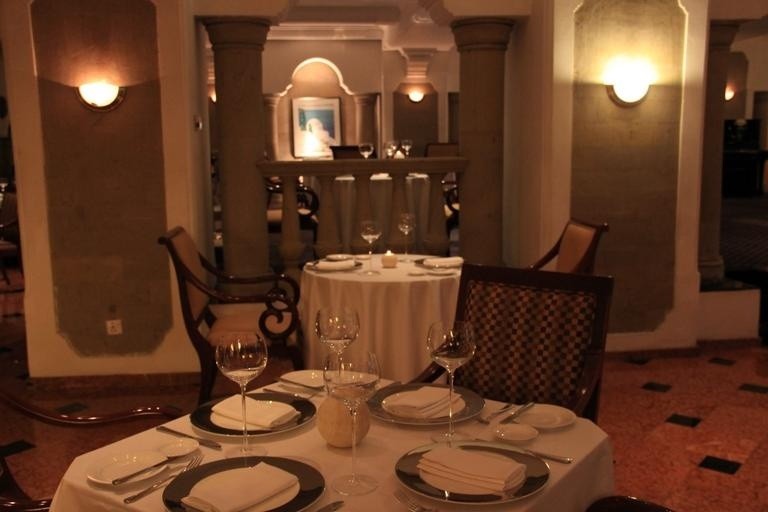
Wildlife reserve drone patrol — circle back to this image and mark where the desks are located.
[335,173,430,253]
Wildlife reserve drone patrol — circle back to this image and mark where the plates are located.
[353,255,372,260]
[307,260,363,272]
[158,438,200,459]
[277,370,378,390]
[408,267,427,275]
[478,403,578,430]
[326,253,357,261]
[163,454,326,509]
[367,381,484,426]
[494,425,538,443]
[86,452,169,484]
[414,257,465,268]
[393,441,551,503]
[190,390,316,439]
[424,268,460,276]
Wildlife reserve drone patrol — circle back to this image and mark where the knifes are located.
[155,424,221,449]
[112,456,191,484]
[498,402,535,427]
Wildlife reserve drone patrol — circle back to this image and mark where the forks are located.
[479,399,520,425]
[124,456,202,504]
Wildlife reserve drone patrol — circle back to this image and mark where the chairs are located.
[531,215,610,273]
[156,226,299,406]
[441,180,460,257]
[586,494,672,511]
[263,152,320,261]
[0,385,184,512]
[424,142,458,156]
[300,254,461,383]
[407,262,616,426]
[329,145,366,160]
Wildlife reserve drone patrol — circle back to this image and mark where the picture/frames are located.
[290,96,341,158]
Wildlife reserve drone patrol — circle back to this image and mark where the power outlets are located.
[104,320,123,335]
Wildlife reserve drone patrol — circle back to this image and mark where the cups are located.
[360,221,384,277]
[215,330,269,457]
[316,307,359,378]
[322,353,382,499]
[358,143,373,161]
[426,318,478,445]
[401,140,413,159]
[398,213,416,263]
[384,143,398,159]
